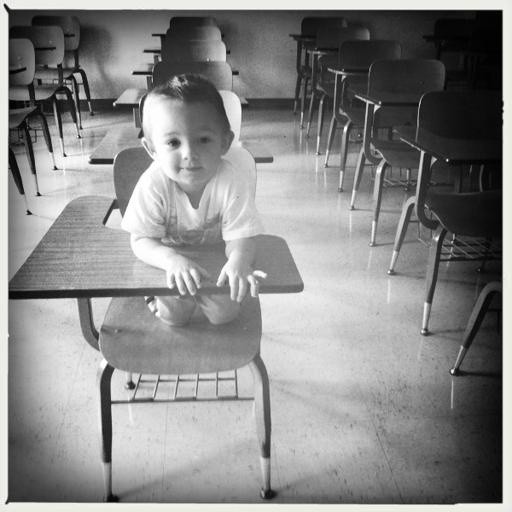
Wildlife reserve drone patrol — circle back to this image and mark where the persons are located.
[121,72,270,327]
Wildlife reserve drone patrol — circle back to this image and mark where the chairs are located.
[8,16,305,497]
[284,16,502,388]
